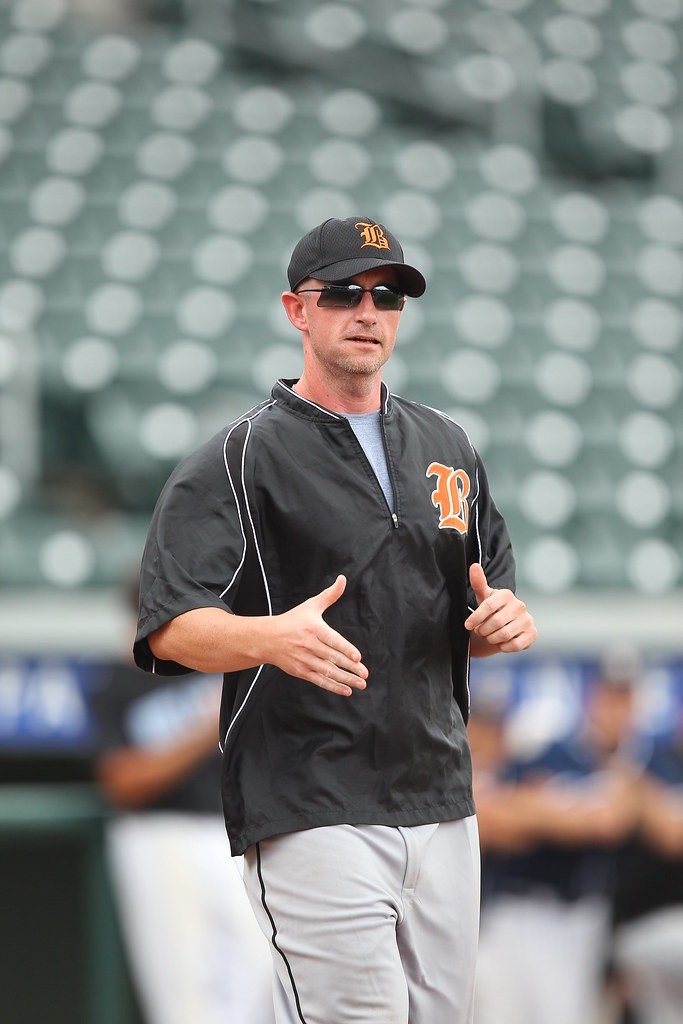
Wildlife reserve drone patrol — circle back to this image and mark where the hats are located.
[288,216,426,298]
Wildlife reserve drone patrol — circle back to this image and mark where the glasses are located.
[296,284,404,311]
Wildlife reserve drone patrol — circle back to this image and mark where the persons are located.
[133,214,539,1024]
[106,654,682,1024]
